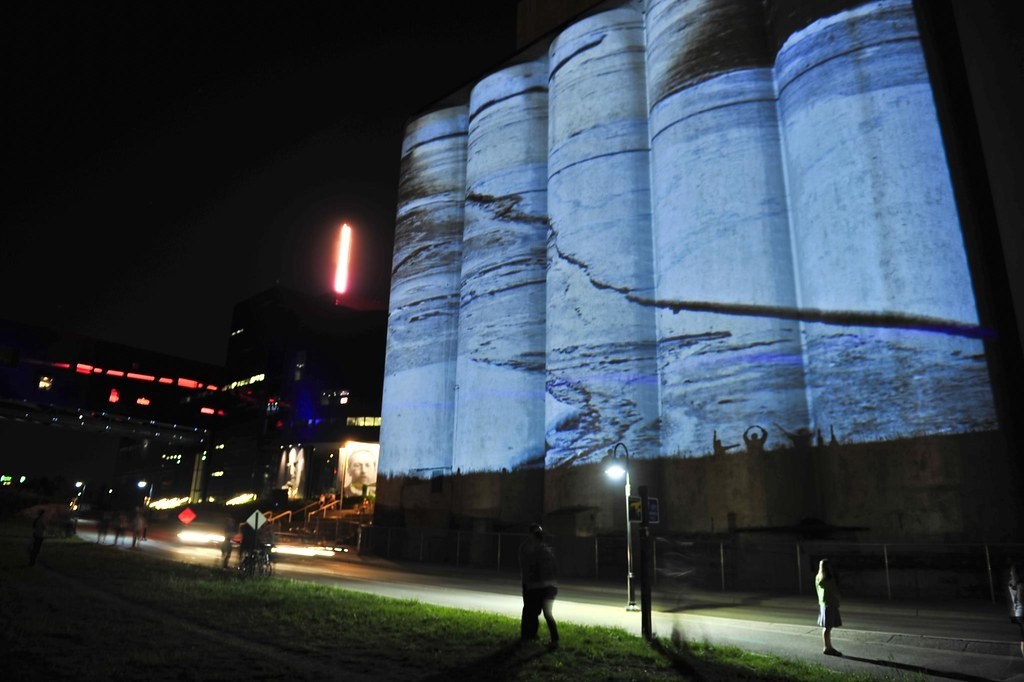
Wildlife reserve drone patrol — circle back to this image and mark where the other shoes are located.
[824,649,842,656]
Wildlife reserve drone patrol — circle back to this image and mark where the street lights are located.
[75,481,86,520]
[137,481,154,541]
[605,442,639,611]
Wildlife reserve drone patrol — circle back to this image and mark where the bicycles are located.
[238,543,274,580]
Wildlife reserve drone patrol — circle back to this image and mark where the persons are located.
[113,511,128,546]
[328,492,336,510]
[353,504,360,515]
[361,498,368,513]
[344,448,377,502]
[318,493,325,509]
[814,558,841,655]
[279,446,304,499]
[95,511,110,544]
[220,516,240,568]
[28,508,47,566]
[517,522,560,649]
[1006,563,1024,658]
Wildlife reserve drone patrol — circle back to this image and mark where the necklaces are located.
[129,513,144,549]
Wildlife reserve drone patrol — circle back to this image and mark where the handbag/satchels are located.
[1009,616,1017,623]
